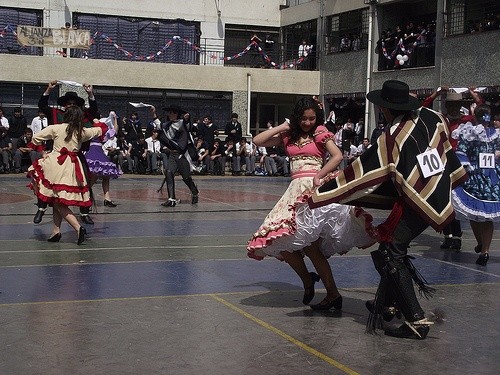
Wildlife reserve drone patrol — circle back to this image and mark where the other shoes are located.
[476,252,489,266]
[80,214,94,225]
[34,210,46,224]
[474,246,482,253]
[310,295,342,315]
[191,194,198,204]
[161,200,176,207]
[440,235,462,249]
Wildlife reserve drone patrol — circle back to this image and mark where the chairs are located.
[0,139,289,174]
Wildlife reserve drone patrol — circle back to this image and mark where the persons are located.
[299,39,311,70]
[26,80,121,245]
[421,87,500,266]
[245,96,377,310]
[377,21,436,70]
[340,33,360,53]
[0,106,48,174]
[468,11,500,33]
[61,22,77,57]
[326,111,384,173]
[108,104,290,207]
[307,80,470,339]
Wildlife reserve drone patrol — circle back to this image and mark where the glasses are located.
[66,102,77,106]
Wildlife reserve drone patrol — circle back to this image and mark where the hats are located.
[56,91,85,108]
[166,106,187,114]
[366,80,423,111]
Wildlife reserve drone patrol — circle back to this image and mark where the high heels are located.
[48,232,61,243]
[77,226,87,245]
[384,319,430,338]
[103,199,117,207]
[303,272,320,304]
[365,300,397,322]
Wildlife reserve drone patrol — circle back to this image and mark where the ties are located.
[151,141,156,154]
